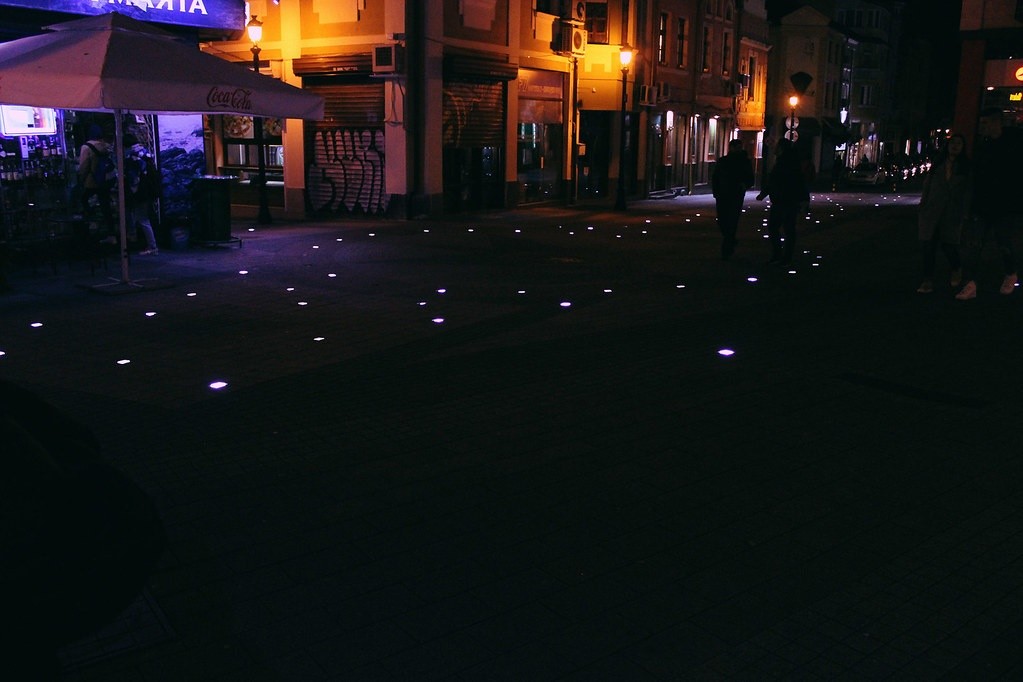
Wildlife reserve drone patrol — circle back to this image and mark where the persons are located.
[954,107,1023,301]
[711,139,755,261]
[121,133,160,257]
[916,133,979,296]
[862,154,868,164]
[76,124,118,245]
[757,138,799,266]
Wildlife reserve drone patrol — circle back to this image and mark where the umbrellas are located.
[0,10,325,283]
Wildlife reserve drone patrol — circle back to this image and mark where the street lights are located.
[612,49,638,214]
[788,93,803,151]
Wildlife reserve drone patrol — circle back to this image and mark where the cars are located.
[844,152,938,188]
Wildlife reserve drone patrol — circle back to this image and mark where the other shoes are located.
[951,270,961,287]
[99,235,118,245]
[917,280,933,293]
[999,272,1017,295]
[955,281,976,300]
[139,248,159,257]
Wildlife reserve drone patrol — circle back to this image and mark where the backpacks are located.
[84,143,117,188]
[134,154,162,203]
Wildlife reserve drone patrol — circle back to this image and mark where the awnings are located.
[824,117,849,136]
[783,118,822,137]
